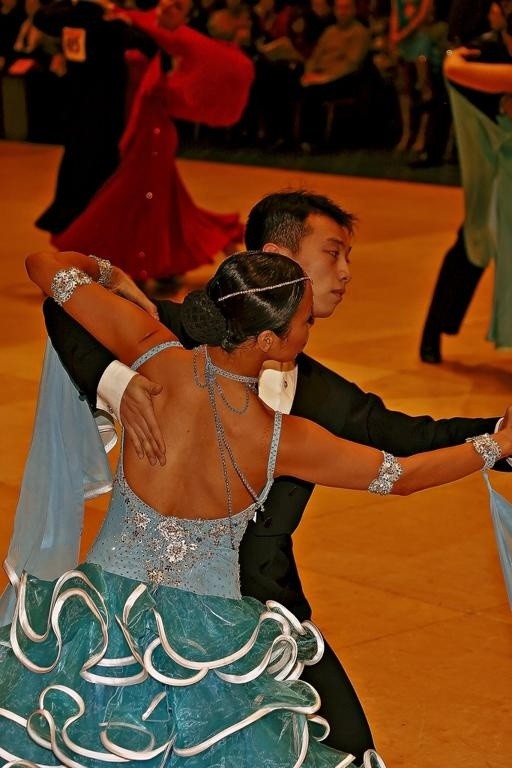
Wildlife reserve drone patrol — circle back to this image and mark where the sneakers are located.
[419,325,441,363]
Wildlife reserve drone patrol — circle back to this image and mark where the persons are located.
[47,0,257,292]
[30,0,179,300]
[0,243,510,768]
[416,0,512,368]
[440,8,511,354]
[38,185,507,768]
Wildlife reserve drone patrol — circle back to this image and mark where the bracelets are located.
[89,252,115,288]
[465,431,504,472]
